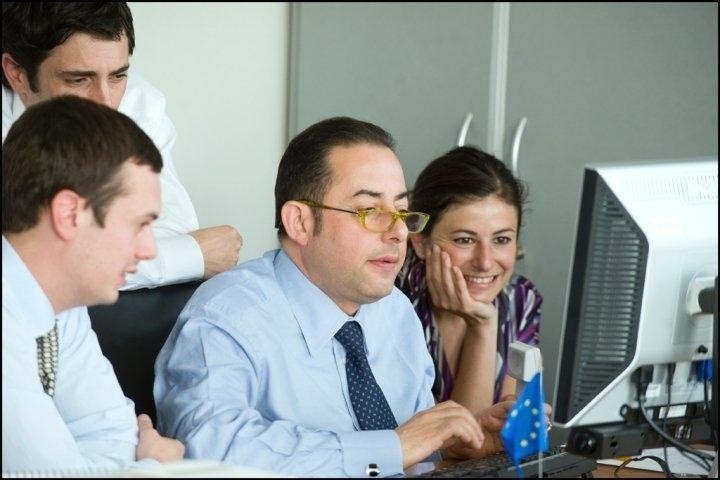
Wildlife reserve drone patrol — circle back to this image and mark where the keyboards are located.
[414,436,598,479]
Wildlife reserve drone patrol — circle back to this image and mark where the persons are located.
[394,144,544,417]
[1,92,186,478]
[1,0,242,294]
[150,115,550,478]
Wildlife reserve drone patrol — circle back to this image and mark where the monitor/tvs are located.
[550,159,719,479]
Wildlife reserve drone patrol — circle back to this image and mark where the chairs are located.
[85,278,211,432]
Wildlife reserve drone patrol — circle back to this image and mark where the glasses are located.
[308,205,430,233]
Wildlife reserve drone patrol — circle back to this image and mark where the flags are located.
[500,373,549,478]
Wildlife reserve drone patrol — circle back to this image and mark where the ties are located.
[36,324,59,398]
[334,321,399,431]
